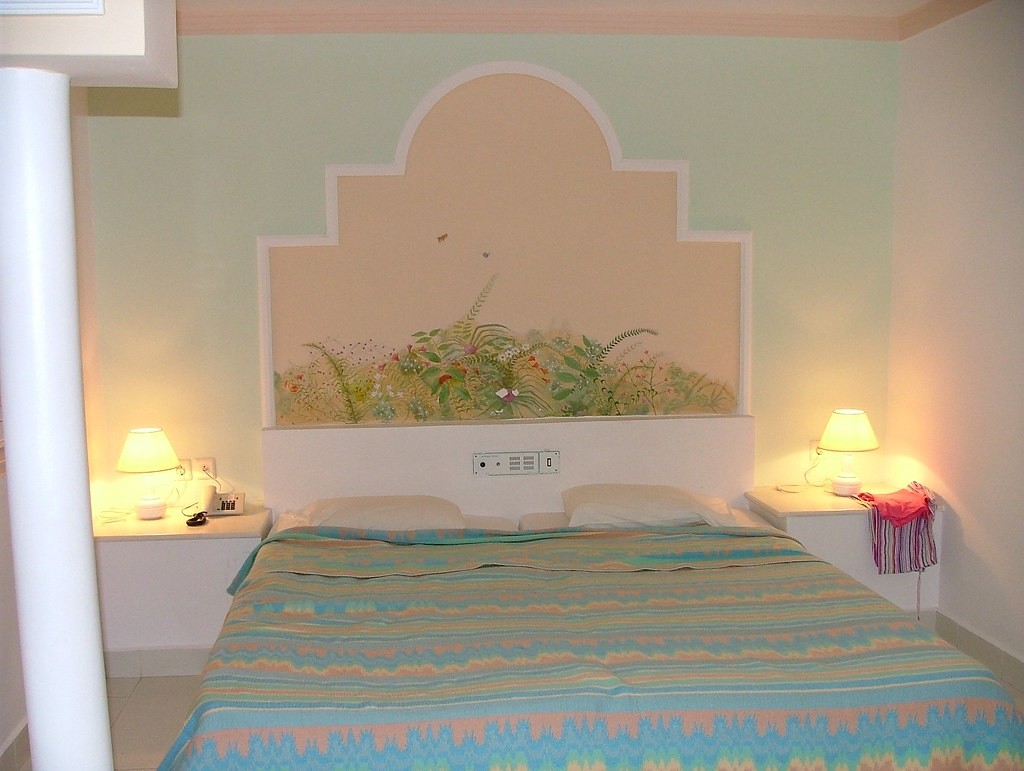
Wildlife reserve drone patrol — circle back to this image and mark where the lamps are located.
[115,428,179,519]
[817,409,880,495]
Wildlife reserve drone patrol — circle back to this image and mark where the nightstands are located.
[748,490,947,613]
[92,506,273,678]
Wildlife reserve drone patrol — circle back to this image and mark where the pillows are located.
[561,484,754,531]
[302,496,464,537]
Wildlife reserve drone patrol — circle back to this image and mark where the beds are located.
[145,529,1024,771]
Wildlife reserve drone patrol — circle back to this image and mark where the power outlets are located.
[194,458,216,480]
[169,460,192,481]
[809,440,826,459]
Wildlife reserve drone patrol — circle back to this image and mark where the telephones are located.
[197,486,246,515]
